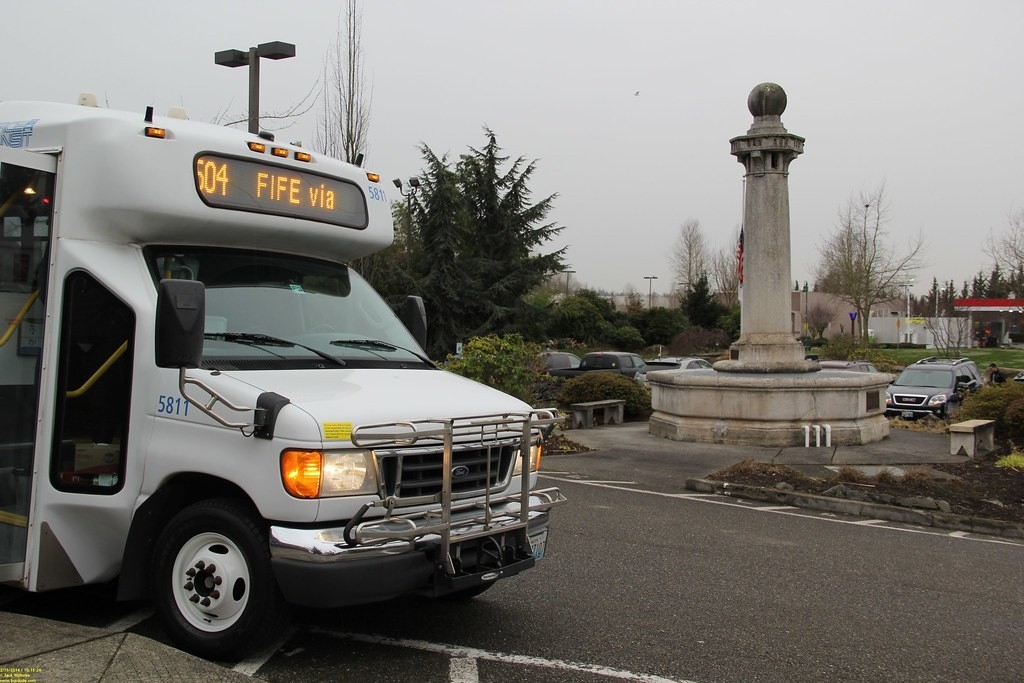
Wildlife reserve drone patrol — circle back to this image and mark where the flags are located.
[737,228,744,287]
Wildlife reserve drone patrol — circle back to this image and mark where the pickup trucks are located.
[543,352,646,382]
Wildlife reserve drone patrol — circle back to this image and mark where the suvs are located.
[886,357,987,423]
[633,357,713,393]
[814,360,892,402]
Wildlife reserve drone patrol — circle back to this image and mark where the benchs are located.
[570,399,626,428]
[950,419,996,457]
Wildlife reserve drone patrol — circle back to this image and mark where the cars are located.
[534,352,582,374]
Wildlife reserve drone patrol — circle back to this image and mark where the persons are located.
[989,364,1005,382]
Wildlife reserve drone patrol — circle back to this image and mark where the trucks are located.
[0,95,568,655]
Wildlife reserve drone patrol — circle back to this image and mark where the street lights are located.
[215,40,298,134]
[848,311,858,338]
[801,279,809,337]
[562,270,576,298]
[941,286,947,315]
[904,276,914,343]
[643,277,658,307]
[679,282,691,302]
